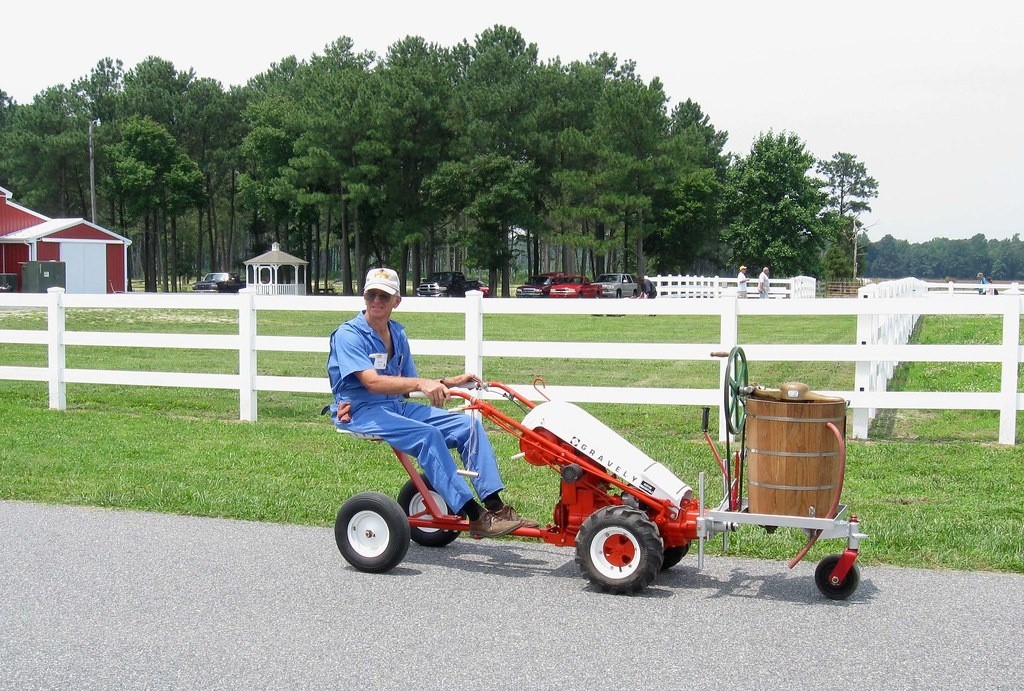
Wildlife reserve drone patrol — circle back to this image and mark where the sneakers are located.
[470,510,522,538]
[492,505,539,528]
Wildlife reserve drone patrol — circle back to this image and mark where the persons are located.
[637,275,657,298]
[977,273,992,295]
[327,268,540,538]
[758,267,769,299]
[737,266,750,298]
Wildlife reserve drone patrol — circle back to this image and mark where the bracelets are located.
[440,378,445,384]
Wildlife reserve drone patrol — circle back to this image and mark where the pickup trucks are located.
[549,275,603,299]
[516,272,564,298]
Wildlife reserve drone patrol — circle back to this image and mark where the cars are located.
[467,280,489,298]
[192,272,245,293]
[591,273,638,297]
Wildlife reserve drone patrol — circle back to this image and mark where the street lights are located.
[88,117,101,226]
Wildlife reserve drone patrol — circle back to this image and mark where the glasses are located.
[364,290,399,303]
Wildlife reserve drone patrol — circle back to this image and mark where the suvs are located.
[416,271,468,298]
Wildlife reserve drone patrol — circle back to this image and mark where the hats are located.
[364,268,400,297]
